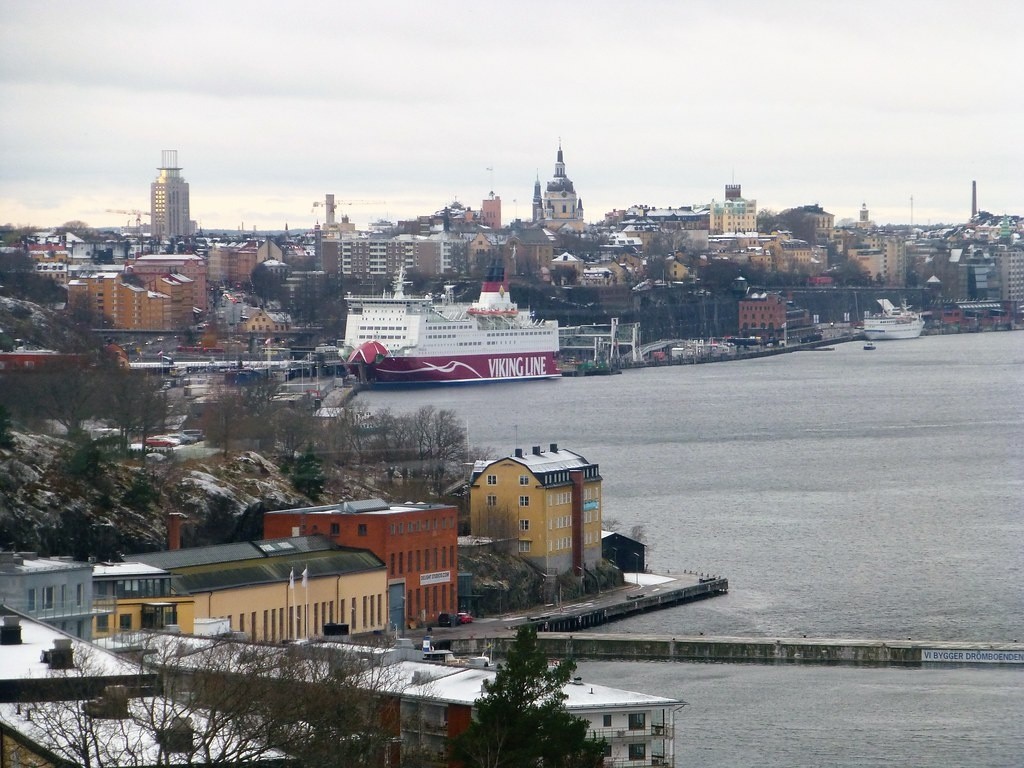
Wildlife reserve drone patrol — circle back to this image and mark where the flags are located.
[301,569,306,587]
[289,571,294,589]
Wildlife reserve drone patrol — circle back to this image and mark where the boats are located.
[864,342,876,350]
[343,265,563,390]
[863,298,925,340]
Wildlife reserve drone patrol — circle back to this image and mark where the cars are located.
[438,613,461,627]
[459,613,474,624]
